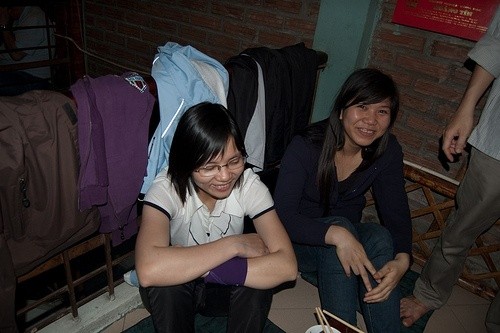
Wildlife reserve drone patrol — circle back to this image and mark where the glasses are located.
[193,155,248,176]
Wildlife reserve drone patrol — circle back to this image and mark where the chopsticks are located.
[315,308,333,333]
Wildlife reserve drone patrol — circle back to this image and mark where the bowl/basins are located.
[305,325,340,333]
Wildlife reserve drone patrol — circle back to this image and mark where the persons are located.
[401,2,500,333]
[273,68,414,333]
[134,102,299,333]
